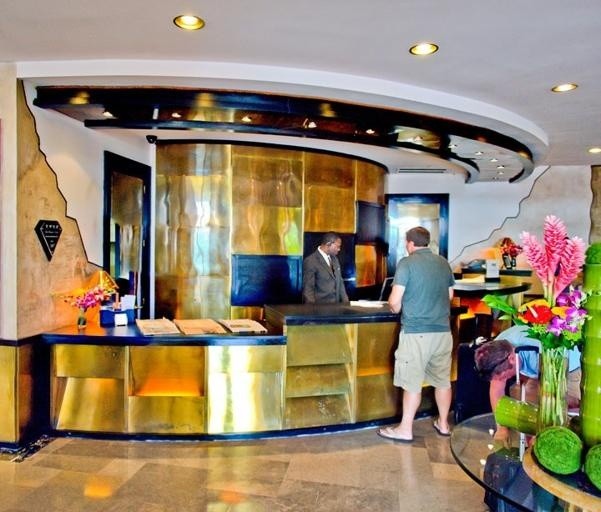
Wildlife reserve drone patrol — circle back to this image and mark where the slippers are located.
[376,426,414,443]
[433,419,452,436]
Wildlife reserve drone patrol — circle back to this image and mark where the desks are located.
[448,410,601,512]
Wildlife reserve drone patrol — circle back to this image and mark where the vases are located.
[536,347,570,432]
[77,309,87,330]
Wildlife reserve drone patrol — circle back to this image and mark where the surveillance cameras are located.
[146,135,157,143]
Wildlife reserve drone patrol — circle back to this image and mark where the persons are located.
[474,324,582,451]
[302,232,349,304]
[376,227,455,441]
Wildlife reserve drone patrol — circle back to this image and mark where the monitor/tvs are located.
[378,277,394,301]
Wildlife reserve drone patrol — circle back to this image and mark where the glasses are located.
[473,354,510,376]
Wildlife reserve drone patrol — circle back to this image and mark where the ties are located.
[328,257,334,275]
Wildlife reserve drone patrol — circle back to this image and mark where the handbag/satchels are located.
[483,445,601,511]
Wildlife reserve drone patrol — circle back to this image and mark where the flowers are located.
[480,214,592,429]
[64,285,112,313]
